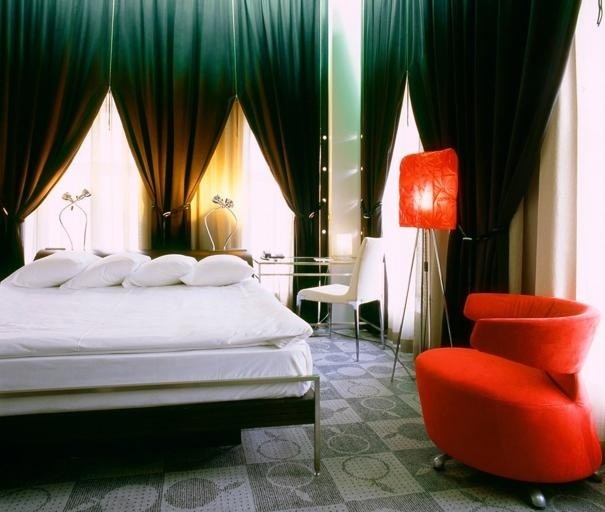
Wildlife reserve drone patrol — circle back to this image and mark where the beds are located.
[0,249,321,477]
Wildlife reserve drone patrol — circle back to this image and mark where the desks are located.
[252,255,356,336]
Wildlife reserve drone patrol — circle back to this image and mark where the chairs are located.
[413,291,603,510]
[296,235,385,361]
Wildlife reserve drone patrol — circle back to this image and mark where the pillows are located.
[178,254,259,287]
[122,253,198,287]
[10,249,103,288]
[58,251,151,288]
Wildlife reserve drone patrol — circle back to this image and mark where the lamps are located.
[58,188,92,251]
[389,145,460,383]
[203,195,239,250]
